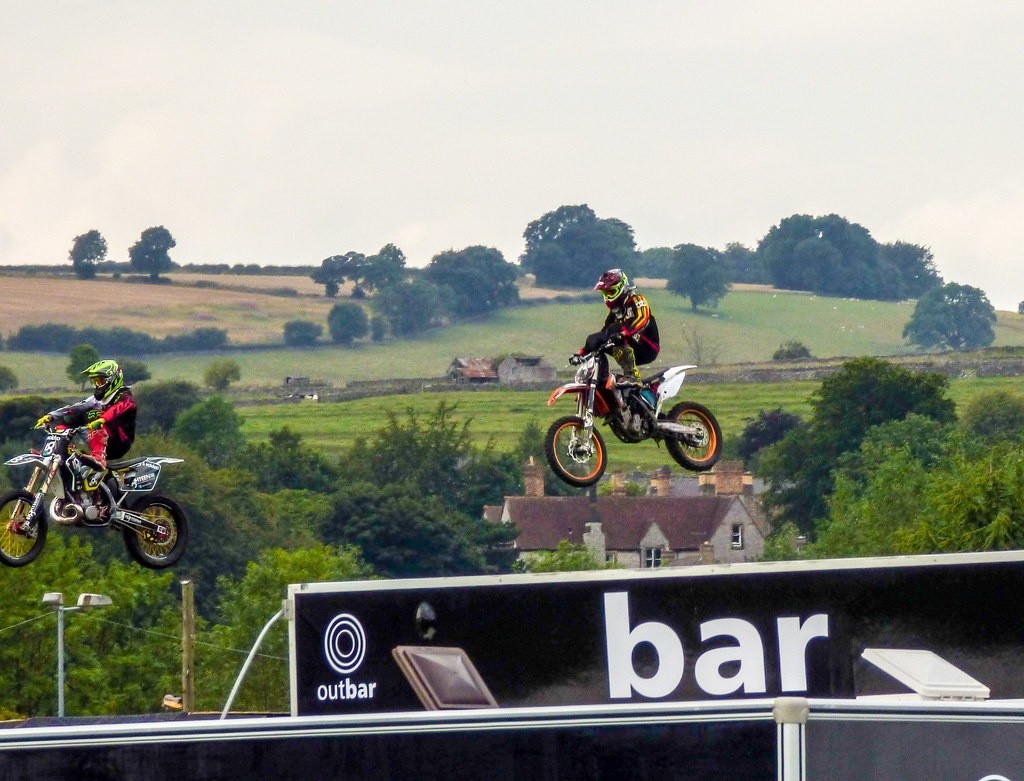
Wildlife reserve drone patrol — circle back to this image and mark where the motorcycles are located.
[0,402,189,571]
[543,329,723,486]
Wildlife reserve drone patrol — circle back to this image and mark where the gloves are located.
[568,354,580,366]
[611,333,623,347]
[87,417,106,430]
[33,414,51,429]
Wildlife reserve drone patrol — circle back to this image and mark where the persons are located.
[569,268,660,389]
[35,360,137,472]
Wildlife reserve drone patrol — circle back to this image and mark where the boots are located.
[614,344,643,390]
[79,428,109,472]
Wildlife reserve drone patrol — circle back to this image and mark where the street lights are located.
[39,592,113,720]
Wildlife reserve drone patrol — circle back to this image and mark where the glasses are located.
[90,376,107,384]
[602,289,615,294]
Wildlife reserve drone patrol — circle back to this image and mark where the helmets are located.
[594,267,629,310]
[80,359,125,401]
